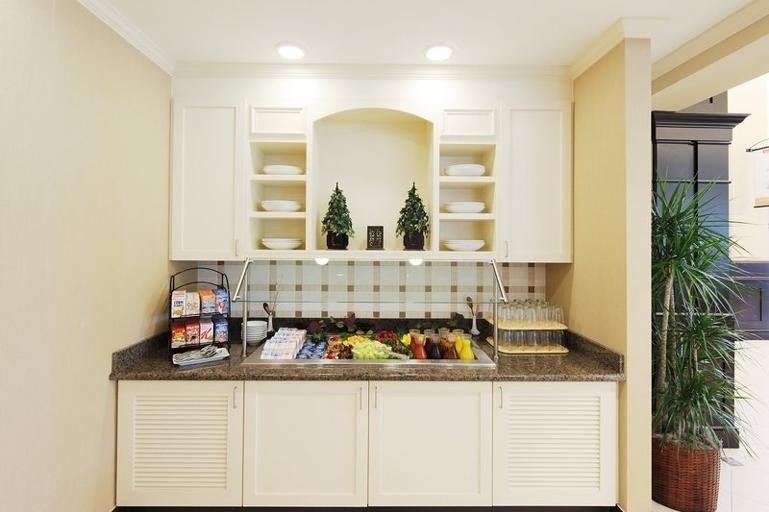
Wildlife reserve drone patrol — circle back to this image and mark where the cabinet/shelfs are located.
[368,382,493,507]
[171,81,243,261]
[652,110,752,450]
[501,80,574,263]
[729,261,769,340]
[243,381,368,507]
[116,380,242,507]
[494,382,619,507]
[243,81,504,261]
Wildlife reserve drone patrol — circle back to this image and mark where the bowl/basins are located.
[240,320,268,347]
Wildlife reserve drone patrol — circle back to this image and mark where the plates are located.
[443,201,485,213]
[445,163,486,176]
[486,336,569,354]
[440,239,485,251]
[261,238,305,249]
[485,319,569,330]
[263,165,302,175]
[261,200,301,211]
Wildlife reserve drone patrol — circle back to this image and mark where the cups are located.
[488,299,566,327]
[492,330,565,351]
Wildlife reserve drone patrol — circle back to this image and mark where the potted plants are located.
[652,169,767,512]
[321,182,356,250]
[395,182,430,250]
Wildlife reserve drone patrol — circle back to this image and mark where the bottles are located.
[442,336,458,359]
[438,328,449,356]
[408,329,420,353]
[412,334,427,359]
[452,329,464,354]
[428,336,442,358]
[423,329,434,356]
[458,334,474,359]
